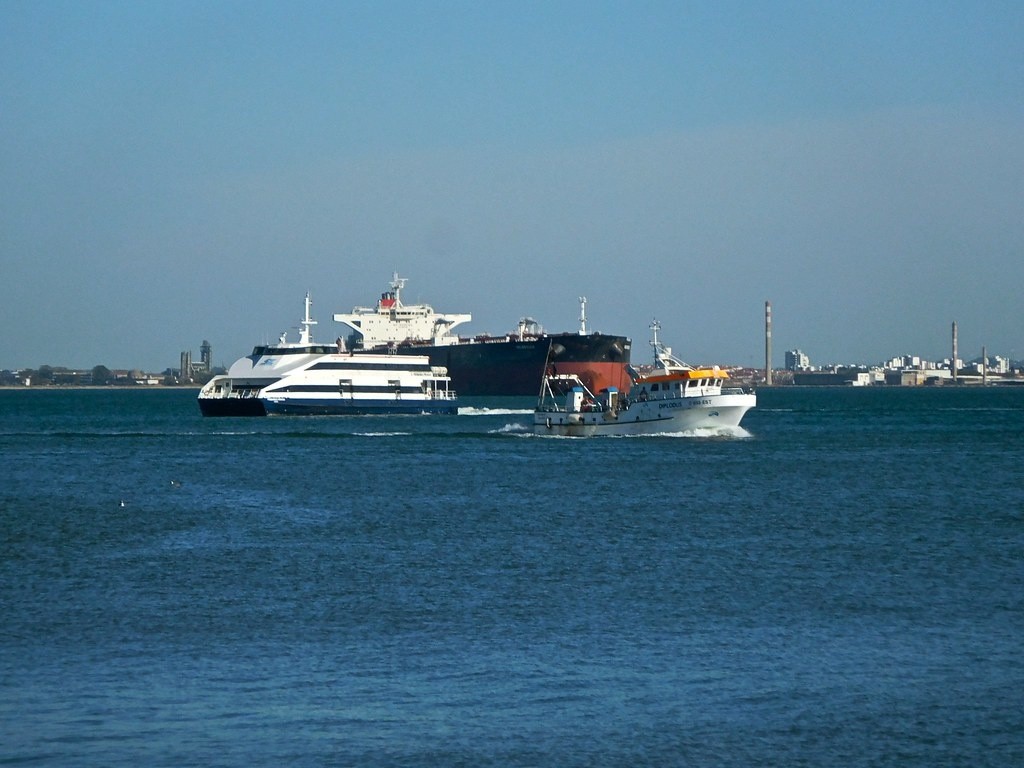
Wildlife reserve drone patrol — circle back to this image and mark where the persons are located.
[640,386,648,402]
[581,396,593,412]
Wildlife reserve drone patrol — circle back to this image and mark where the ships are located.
[198,267,632,416]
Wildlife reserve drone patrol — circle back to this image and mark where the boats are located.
[254,335,460,416]
[531,316,757,436]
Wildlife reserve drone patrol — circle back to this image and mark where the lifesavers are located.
[640,390,648,400]
